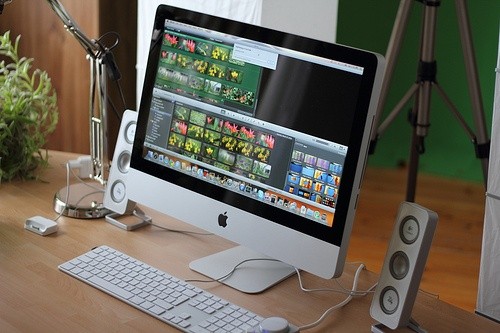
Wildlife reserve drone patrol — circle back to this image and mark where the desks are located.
[0,148,500,332]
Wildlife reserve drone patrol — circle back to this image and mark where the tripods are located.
[367,1,491,202]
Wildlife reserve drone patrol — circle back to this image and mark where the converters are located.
[77,155,94,178]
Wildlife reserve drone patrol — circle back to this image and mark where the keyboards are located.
[56,244,264,333]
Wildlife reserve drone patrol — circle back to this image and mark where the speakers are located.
[102,108,152,230]
[367,201,438,332]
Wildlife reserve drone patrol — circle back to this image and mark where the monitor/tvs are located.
[125,1,386,295]
